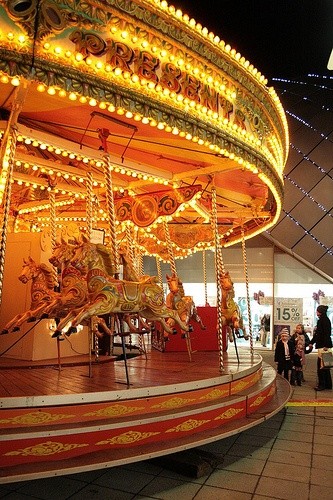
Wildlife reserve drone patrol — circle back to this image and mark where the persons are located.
[274,305,333,391]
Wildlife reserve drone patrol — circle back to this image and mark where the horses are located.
[0,234,207,341]
[216,270,249,343]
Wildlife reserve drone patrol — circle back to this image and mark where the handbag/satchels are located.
[320,349,333,369]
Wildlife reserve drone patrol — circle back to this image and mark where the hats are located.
[317,305,328,314]
[281,328,289,336]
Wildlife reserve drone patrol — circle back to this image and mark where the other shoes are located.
[290,383,296,386]
[302,379,305,382]
[314,387,324,391]
[297,383,301,386]
